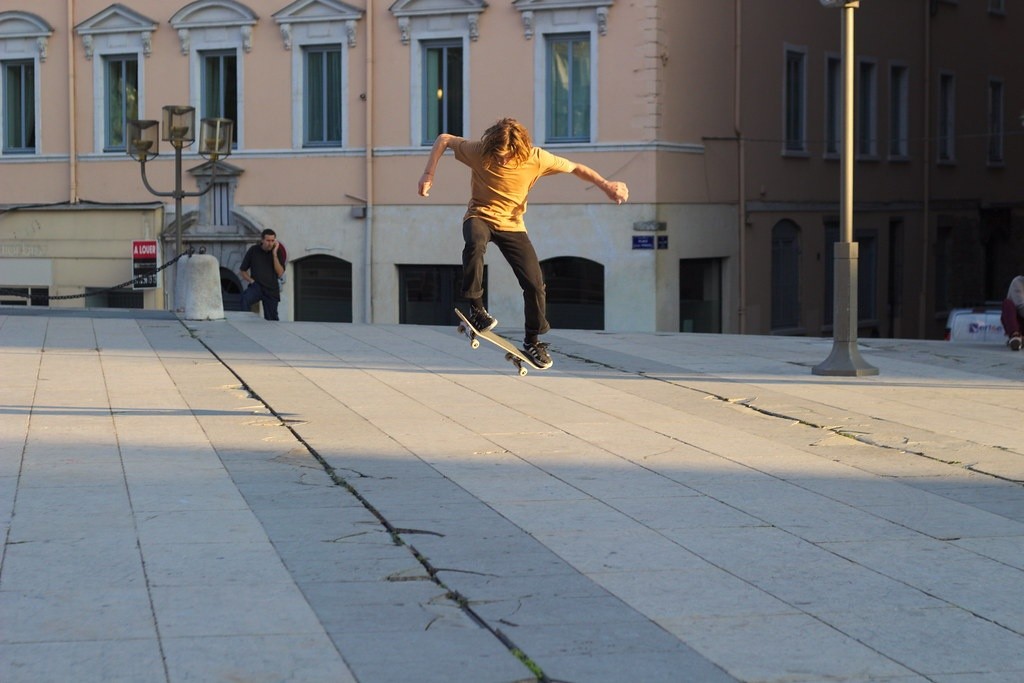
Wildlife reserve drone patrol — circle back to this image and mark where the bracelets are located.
[424,172,434,176]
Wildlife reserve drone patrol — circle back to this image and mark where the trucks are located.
[946,300,1010,345]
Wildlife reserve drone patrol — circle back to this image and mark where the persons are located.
[1001,276,1024,351]
[418,118,629,367]
[239,228,285,320]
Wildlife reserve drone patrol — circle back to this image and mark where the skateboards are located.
[454,307,554,377]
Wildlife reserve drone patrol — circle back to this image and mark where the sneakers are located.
[523,340,553,367]
[469,306,498,334]
